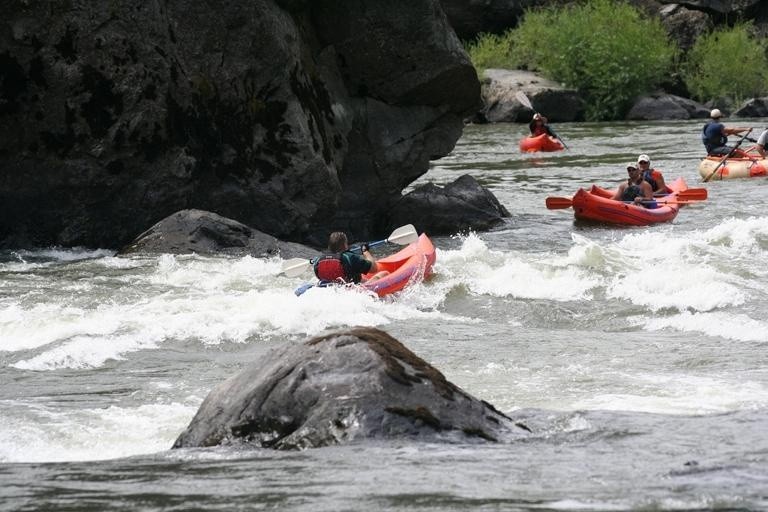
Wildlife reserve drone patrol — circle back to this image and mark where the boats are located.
[295,232,437,301]
[591,175,691,209]
[572,188,678,227]
[519,133,565,153]
[699,153,768,181]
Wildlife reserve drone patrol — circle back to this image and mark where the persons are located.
[312,231,391,287]
[636,154,666,198]
[529,113,558,139]
[756,128,768,159]
[606,162,653,209]
[702,109,752,158]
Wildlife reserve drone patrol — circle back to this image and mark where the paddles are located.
[546,197,692,209]
[654,189,707,200]
[282,222,419,278]
[516,92,569,149]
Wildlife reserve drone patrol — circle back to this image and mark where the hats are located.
[638,154,650,164]
[626,162,640,170]
[710,108,722,118]
[533,112,547,124]
